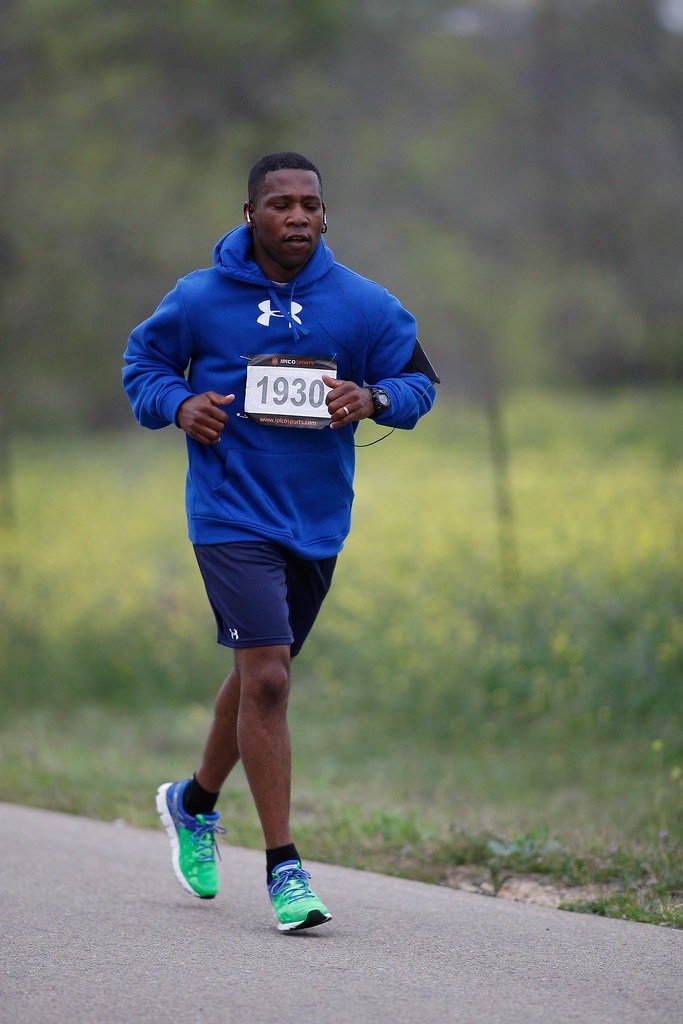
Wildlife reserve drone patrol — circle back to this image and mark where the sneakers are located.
[266,860,332,932]
[155,776,222,899]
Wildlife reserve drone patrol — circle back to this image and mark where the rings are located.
[343,407,350,415]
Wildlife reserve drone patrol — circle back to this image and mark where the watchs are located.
[367,386,391,419]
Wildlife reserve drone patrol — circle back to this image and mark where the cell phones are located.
[412,338,440,384]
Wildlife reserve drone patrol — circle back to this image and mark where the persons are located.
[122,151,443,932]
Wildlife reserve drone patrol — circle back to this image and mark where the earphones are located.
[245,200,253,223]
[321,201,327,233]
